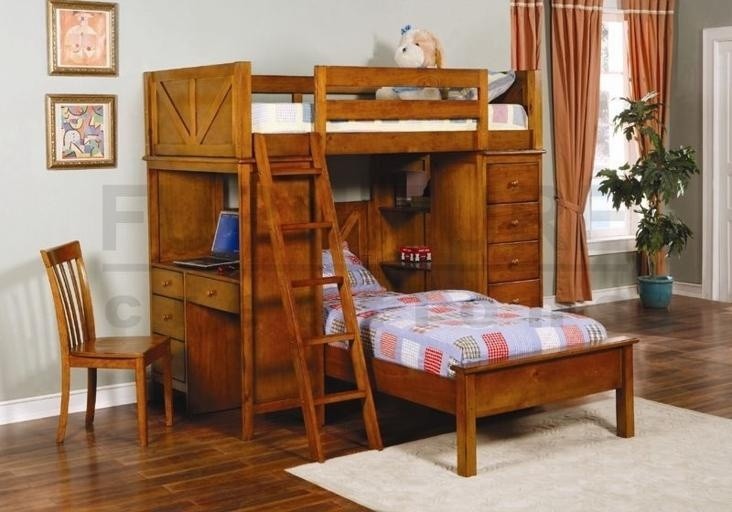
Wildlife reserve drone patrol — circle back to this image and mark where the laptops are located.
[172,208,240,270]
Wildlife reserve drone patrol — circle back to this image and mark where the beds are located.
[142,63,641,475]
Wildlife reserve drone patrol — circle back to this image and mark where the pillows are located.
[317,241,379,295]
[442,64,517,103]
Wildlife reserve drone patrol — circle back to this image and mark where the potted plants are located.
[595,88,703,307]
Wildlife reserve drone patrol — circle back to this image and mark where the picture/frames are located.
[45,92,120,169]
[46,1,119,77]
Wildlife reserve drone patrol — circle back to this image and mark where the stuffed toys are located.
[376,25,442,100]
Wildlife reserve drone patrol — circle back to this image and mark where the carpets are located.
[282,391,731,509]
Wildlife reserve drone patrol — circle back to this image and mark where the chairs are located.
[39,239,178,446]
